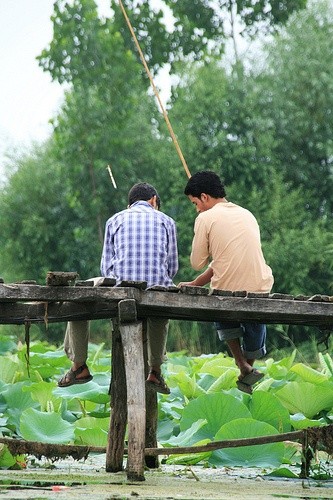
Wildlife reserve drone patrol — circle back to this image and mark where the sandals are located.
[58,363,93,387]
[145,370,171,395]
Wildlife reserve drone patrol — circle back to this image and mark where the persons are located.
[57,182,178,395]
[177,171,274,395]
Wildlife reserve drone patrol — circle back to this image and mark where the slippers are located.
[238,369,265,385]
[236,380,253,395]
